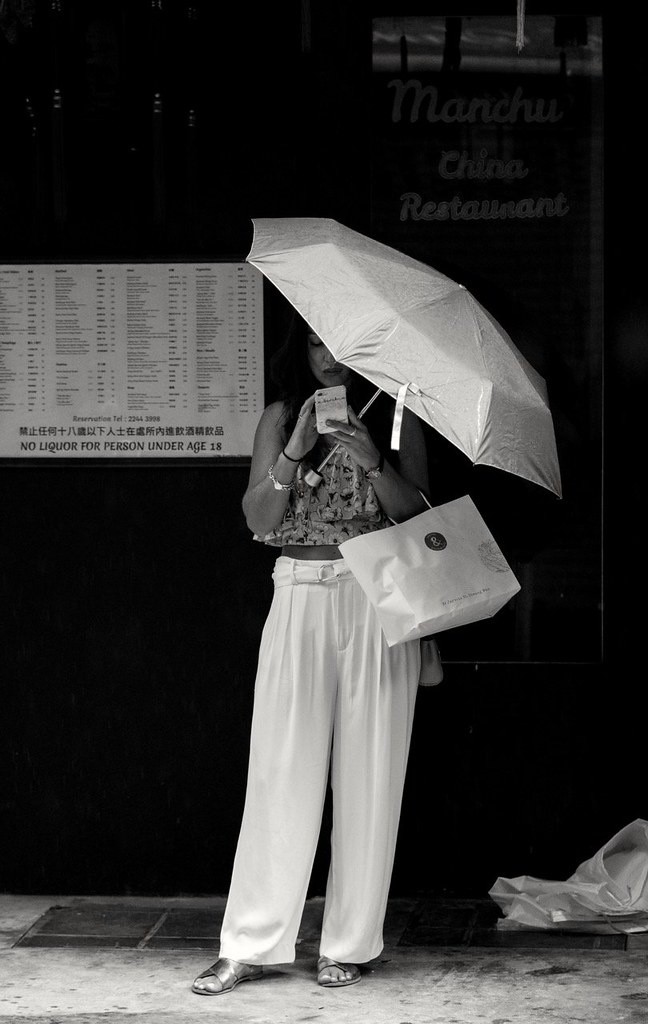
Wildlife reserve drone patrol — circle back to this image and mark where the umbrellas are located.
[246,214,563,499]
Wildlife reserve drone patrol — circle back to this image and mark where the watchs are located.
[266,463,298,493]
[364,456,385,482]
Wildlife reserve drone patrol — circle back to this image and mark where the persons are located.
[184,320,418,997]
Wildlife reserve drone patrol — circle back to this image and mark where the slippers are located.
[190,959,265,997]
[317,955,362,986]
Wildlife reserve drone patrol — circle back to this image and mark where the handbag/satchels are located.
[336,493,522,648]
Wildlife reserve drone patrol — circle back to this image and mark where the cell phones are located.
[315,385,349,434]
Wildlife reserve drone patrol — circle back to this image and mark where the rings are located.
[350,428,357,436]
[298,415,303,419]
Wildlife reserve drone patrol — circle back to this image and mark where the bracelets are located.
[282,449,303,462]
